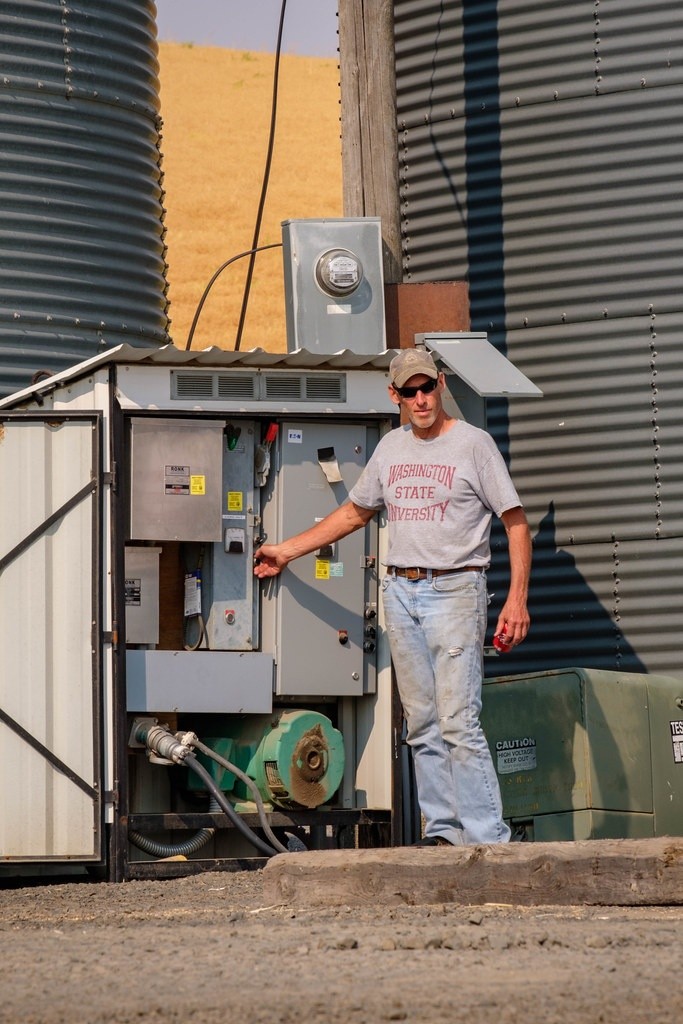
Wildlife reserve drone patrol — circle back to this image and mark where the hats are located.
[389,348,439,388]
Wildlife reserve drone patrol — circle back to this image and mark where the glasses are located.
[391,370,439,399]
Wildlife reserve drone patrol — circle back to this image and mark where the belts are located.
[386,564,483,581]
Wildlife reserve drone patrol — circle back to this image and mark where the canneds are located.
[493,623,513,653]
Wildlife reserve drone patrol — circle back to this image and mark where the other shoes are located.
[412,837,453,847]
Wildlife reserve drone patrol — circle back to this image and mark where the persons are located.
[253,348,532,845]
